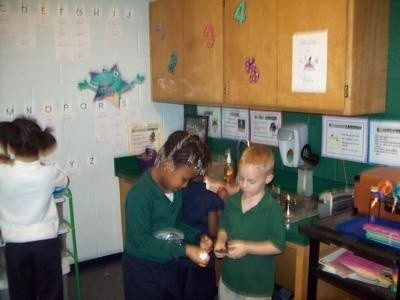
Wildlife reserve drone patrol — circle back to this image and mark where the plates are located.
[299,208,400,300]
[278,0,390,116]
[223,0,278,112]
[182,0,223,108]
[149,0,182,105]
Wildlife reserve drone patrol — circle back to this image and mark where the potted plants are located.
[184,115,209,143]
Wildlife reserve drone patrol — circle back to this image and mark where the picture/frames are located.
[114,151,356,299]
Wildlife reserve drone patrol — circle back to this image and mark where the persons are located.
[179,168,225,298]
[216,144,287,300]
[0,117,69,300]
[120,131,212,297]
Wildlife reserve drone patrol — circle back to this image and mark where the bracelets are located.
[136,156,155,170]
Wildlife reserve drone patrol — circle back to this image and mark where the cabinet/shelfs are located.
[297,163,314,197]
[223,148,234,184]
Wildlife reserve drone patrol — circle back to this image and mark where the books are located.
[0,257,74,300]
[0,197,66,242]
[0,224,69,281]
